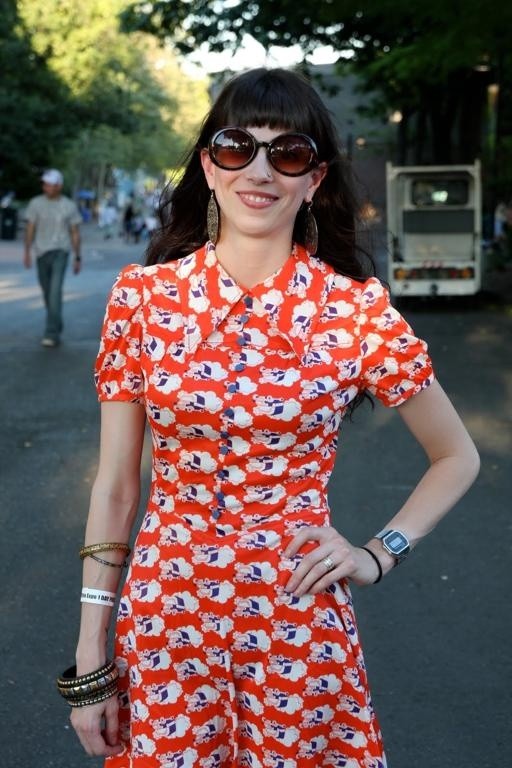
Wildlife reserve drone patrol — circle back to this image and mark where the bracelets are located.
[79,543,131,568]
[57,660,121,708]
[81,585,116,608]
[357,546,382,584]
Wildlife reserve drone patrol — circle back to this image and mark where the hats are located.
[41,169,63,185]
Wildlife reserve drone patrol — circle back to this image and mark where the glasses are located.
[207,127,320,176]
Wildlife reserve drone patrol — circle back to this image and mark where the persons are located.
[56,67,481,768]
[98,171,175,245]
[23,168,82,348]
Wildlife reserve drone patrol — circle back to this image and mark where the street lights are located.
[477,49,506,159]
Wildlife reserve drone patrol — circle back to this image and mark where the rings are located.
[323,557,335,570]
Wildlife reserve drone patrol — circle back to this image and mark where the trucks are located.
[382,158,483,310]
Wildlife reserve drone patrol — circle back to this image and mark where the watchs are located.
[374,529,410,568]
